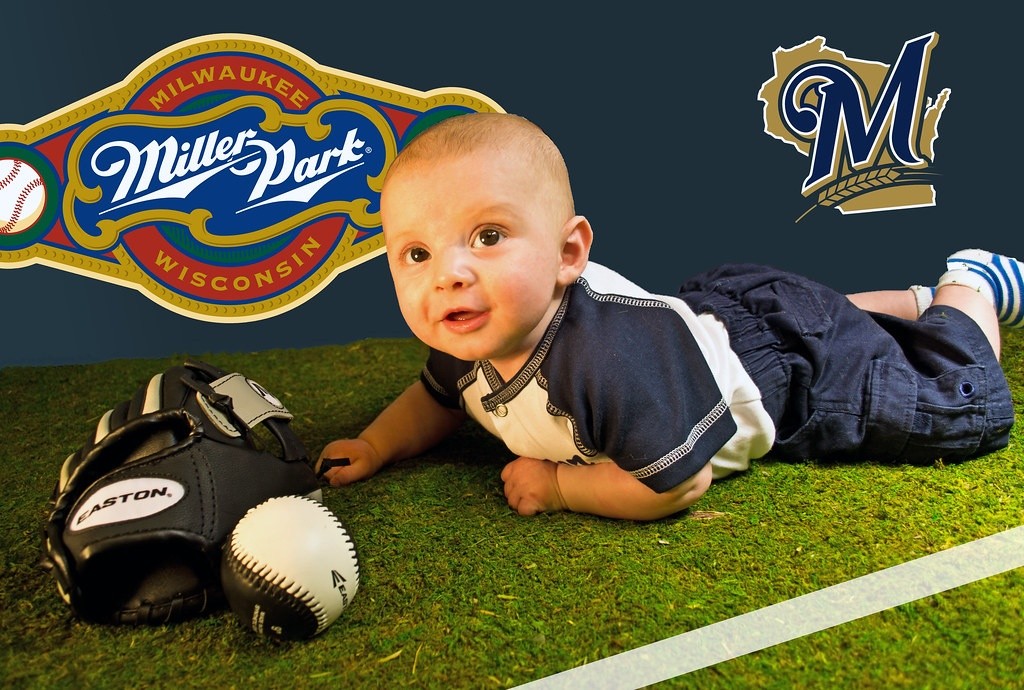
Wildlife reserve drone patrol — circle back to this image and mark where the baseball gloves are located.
[40,357,325,627]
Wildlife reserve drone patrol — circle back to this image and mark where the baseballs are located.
[221,493,364,640]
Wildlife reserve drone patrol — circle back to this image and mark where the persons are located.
[312,110,1024,523]
